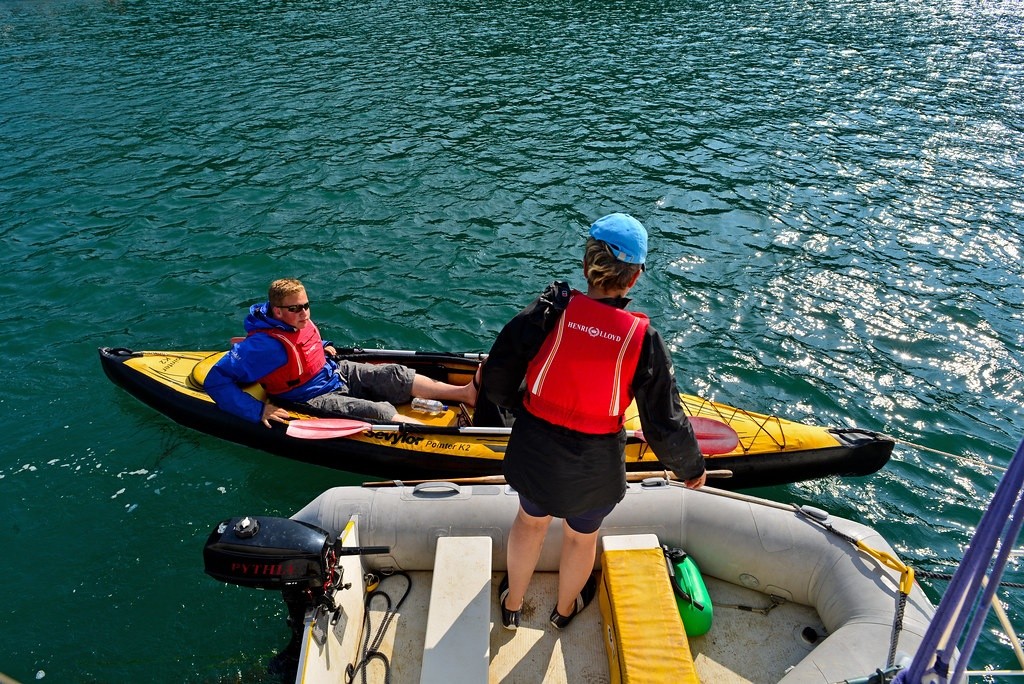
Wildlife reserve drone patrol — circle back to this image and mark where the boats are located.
[202,476,967,684]
[98,340,896,492]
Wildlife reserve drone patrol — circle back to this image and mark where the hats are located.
[589,213,648,272]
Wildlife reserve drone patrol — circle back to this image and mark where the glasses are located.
[277,301,310,313]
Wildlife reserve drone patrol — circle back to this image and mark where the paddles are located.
[229,335,489,360]
[283,414,741,456]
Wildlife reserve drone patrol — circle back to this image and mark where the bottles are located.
[411,398,449,416]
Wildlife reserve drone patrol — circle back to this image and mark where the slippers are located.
[457,403,473,427]
[473,357,486,408]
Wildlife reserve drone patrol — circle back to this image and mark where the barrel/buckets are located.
[668,546,713,637]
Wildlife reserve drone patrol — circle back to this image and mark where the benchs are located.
[420,536,494,684]
[599,534,702,684]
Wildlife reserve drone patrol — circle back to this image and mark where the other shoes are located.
[550,575,596,629]
[498,574,522,630]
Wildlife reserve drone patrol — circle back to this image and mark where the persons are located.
[202,277,483,428]
[479,213,707,631]
